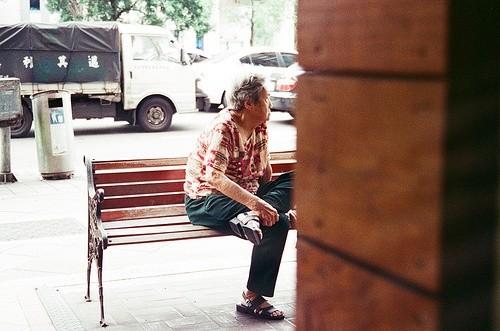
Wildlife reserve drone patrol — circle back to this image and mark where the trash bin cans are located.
[30,89,76,180]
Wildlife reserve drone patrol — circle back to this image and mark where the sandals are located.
[236,291,284,319]
[229,212,262,246]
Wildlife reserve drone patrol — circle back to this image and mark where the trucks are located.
[0,22,199,137]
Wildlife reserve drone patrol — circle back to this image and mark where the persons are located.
[181,74,295,321]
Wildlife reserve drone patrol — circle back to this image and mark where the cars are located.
[196,50,297,112]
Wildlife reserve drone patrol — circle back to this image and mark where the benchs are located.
[83,150,297,327]
